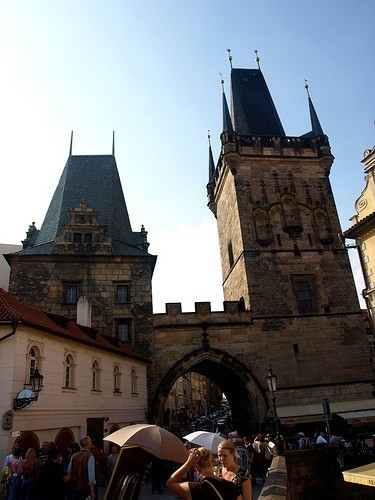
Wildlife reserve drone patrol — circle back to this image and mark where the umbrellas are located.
[182,428,240,458]
[103,419,189,464]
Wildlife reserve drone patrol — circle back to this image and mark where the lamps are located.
[29,366,44,402]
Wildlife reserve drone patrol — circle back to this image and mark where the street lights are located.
[264,365,283,453]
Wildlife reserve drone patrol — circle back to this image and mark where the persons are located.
[1,430,375,500]
